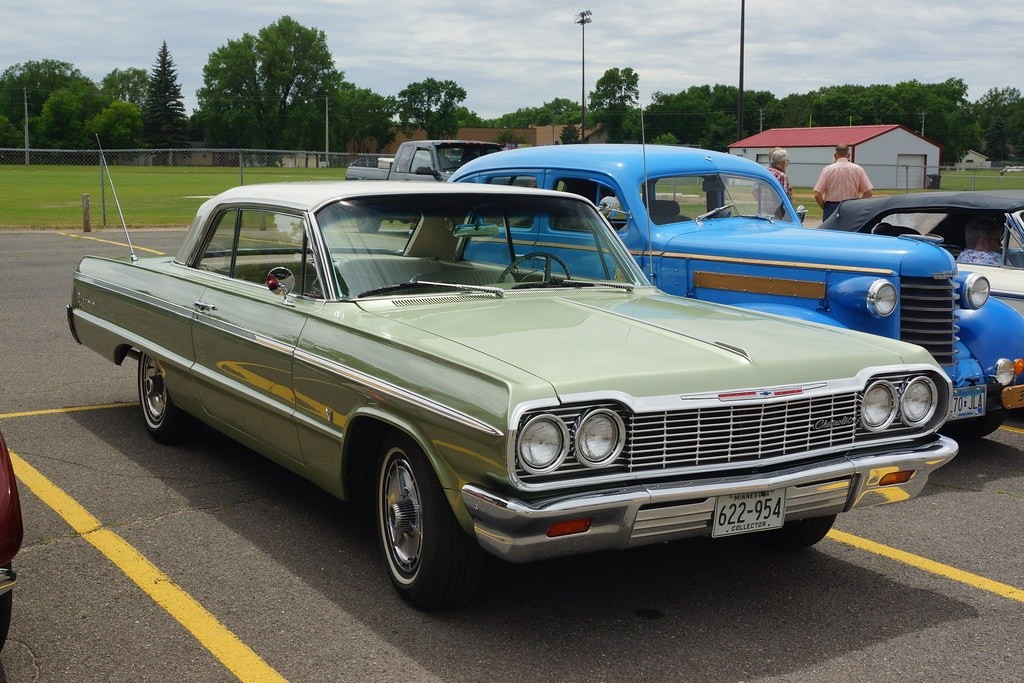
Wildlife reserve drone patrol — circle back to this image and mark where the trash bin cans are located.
[926,174,942,189]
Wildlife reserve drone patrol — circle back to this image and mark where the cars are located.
[446,143,1023,446]
[65,181,960,610]
[816,190,1023,314]
[349,157,377,168]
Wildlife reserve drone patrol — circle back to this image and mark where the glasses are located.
[981,235,1004,241]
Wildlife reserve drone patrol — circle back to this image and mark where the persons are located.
[751,147,793,222]
[813,142,874,223]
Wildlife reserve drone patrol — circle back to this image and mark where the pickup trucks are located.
[345,140,537,233]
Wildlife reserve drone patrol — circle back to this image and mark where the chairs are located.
[309,273,385,298]
[214,261,317,293]
[342,259,447,286]
[410,266,515,290]
[648,199,692,225]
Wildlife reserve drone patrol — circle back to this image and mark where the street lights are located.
[915,131,924,136]
[572,9,593,142]
